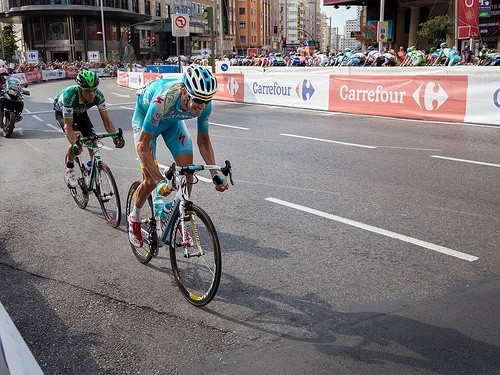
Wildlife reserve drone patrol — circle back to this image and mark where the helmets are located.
[76,70,100,90]
[0,67,8,74]
[232,42,496,59]
[181,64,218,101]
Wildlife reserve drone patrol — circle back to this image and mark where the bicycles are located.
[65,128,125,228]
[125,160,234,307]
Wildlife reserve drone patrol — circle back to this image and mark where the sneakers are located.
[178,224,193,247]
[64,164,79,187]
[128,213,144,248]
[100,188,111,200]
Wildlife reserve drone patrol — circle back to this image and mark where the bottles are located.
[160,202,173,231]
[153,196,164,227]
[85,161,92,180]
[82,164,87,178]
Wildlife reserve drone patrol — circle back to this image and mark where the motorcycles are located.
[0,77,30,137]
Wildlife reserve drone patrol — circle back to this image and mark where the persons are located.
[128,65,229,247]
[53,70,125,189]
[223,41,500,66]
[0,68,23,128]
[0,56,211,84]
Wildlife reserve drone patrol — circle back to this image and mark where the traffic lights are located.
[152,36,155,46]
[283,38,286,47]
[146,38,150,46]
[274,26,277,34]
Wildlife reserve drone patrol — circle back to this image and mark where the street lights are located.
[333,27,338,49]
[309,1,316,41]
[325,17,331,48]
[97,32,106,60]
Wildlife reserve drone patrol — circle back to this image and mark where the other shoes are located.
[17,114,23,122]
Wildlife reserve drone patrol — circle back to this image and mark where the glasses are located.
[191,98,212,105]
